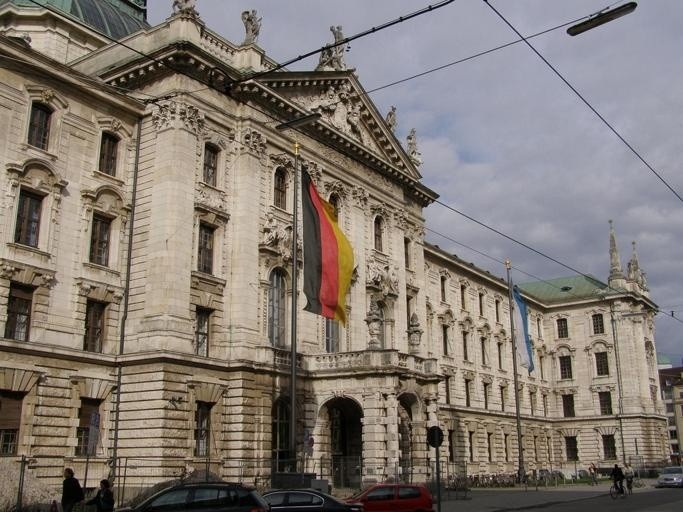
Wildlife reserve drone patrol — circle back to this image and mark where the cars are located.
[341,483,436,512]
[657,466,682,490]
[260,483,365,512]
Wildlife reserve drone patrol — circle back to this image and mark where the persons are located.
[80,479,114,512]
[59,468,83,512]
[608,464,625,496]
[623,462,633,488]
[589,462,598,486]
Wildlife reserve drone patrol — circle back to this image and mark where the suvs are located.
[113,479,270,512]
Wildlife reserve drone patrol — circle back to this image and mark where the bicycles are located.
[453,474,521,489]
[609,477,627,500]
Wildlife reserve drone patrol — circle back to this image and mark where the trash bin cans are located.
[311,480,328,494]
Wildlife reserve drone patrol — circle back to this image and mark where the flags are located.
[510,283,533,373]
[298,159,354,327]
